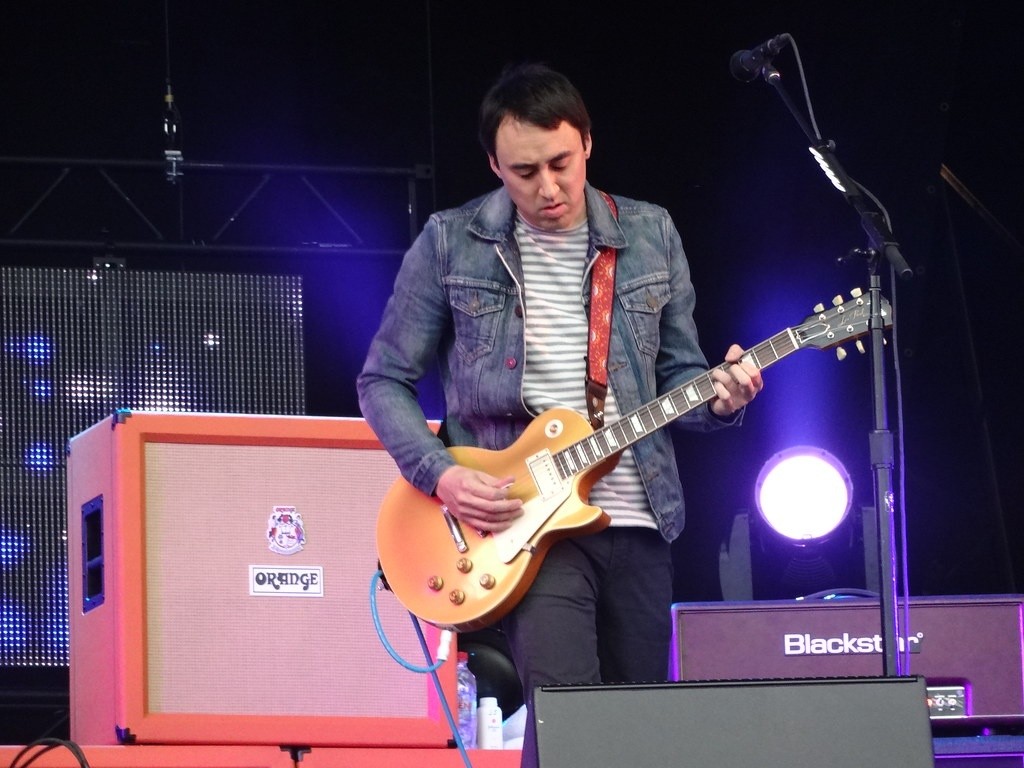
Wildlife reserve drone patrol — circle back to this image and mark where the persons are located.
[357,68,764,768]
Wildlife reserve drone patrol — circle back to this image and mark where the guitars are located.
[374,284,895,634]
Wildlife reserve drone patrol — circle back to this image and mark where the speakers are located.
[520,675,936,767]
[667,589,1023,737]
[66,410,461,748]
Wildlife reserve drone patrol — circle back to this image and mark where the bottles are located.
[456,651,477,749]
[476,697,503,750]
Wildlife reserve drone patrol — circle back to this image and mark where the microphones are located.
[729,32,789,82]
